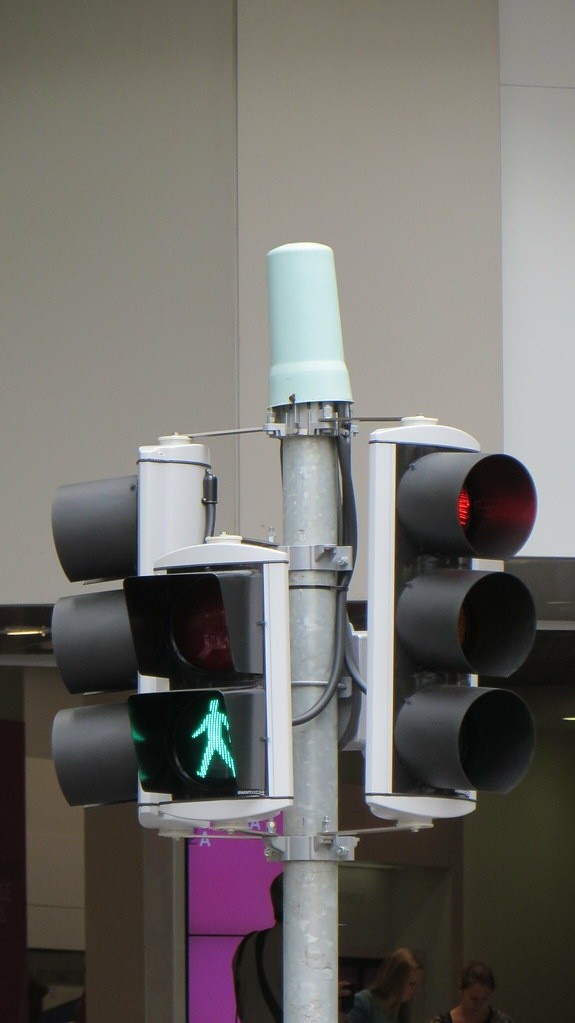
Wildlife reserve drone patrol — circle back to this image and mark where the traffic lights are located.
[117,543,297,835]
[48,432,212,830]
[362,416,539,830]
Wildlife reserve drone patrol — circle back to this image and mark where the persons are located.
[345,947,424,1023]
[431,959,515,1023]
[231,872,352,1023]
